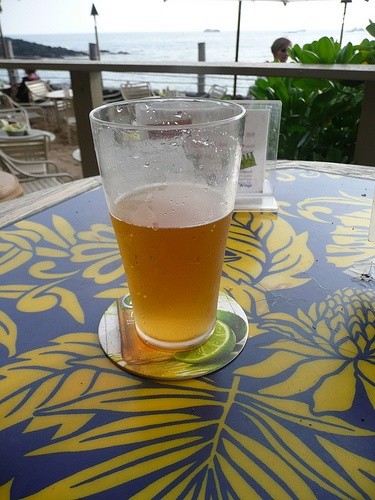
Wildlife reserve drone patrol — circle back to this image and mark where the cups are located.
[89,97,246,353]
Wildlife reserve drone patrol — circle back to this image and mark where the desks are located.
[0,129,56,142]
[46,90,74,98]
[0,160,375,500]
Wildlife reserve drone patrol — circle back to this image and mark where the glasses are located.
[280,48,286,53]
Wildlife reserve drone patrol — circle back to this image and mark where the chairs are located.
[0,79,227,196]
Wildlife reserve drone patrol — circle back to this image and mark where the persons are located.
[12,67,40,103]
[262,38,293,63]
